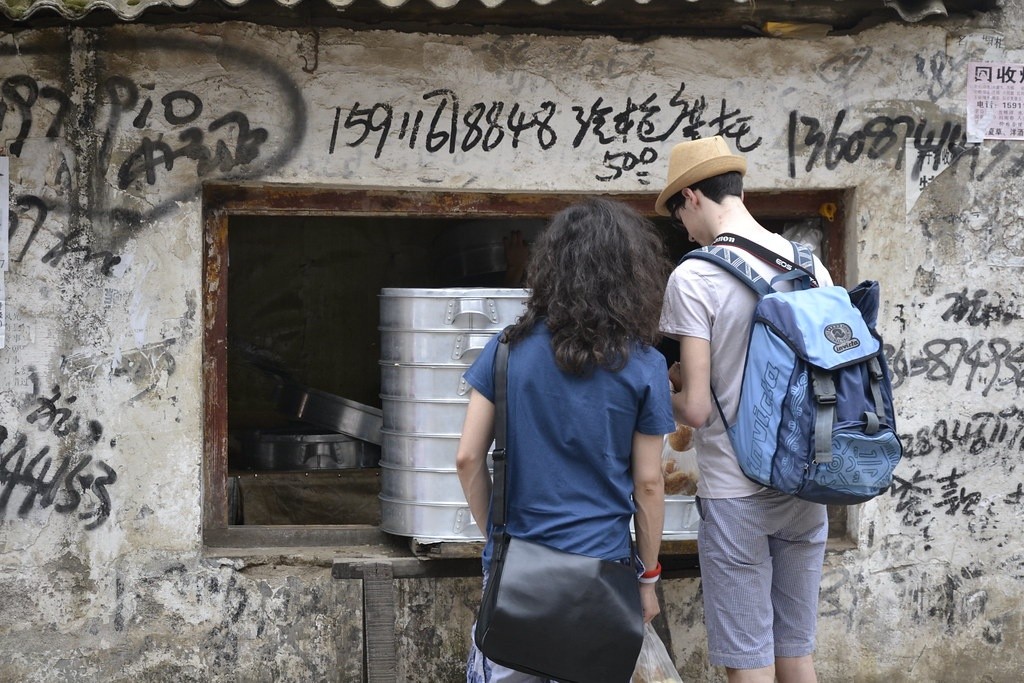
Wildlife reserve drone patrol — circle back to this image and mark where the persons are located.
[654,136,834,683]
[454,199,677,683]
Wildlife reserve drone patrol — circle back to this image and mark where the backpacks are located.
[678,238,903,505]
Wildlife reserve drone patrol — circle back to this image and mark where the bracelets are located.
[669,389,677,393]
[638,561,661,584]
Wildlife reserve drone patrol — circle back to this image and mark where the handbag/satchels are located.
[630,621,684,683]
[476,538,646,683]
[661,378,701,497]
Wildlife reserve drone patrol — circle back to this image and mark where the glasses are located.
[671,198,687,233]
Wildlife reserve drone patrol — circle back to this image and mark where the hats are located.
[655,135,747,216]
